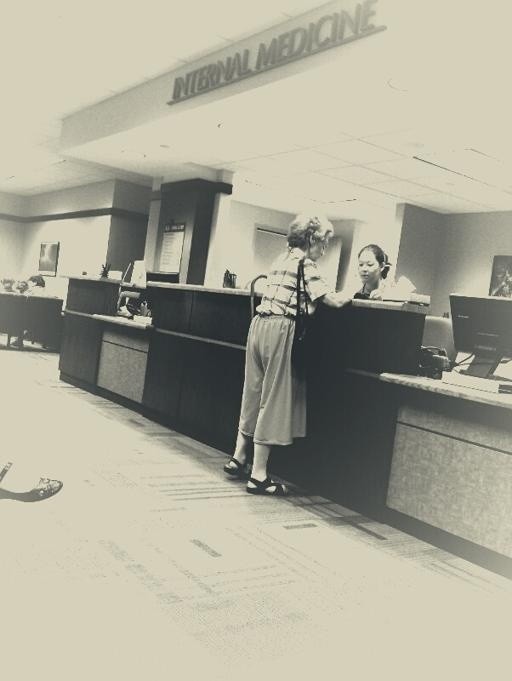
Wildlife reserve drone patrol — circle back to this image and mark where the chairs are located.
[422,314,458,363]
[0,291,64,353]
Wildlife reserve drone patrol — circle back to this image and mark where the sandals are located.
[223,456,253,481]
[246,476,293,497]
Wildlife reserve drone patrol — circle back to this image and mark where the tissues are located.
[381,275,431,307]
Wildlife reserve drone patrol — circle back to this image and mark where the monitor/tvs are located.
[448,293,512,383]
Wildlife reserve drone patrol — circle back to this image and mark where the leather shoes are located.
[0,461,63,502]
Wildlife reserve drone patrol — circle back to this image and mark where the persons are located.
[223,211,364,499]
[354,242,398,305]
[10,275,56,348]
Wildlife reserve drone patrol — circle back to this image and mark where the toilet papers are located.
[128,259,147,289]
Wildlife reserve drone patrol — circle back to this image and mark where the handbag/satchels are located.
[293,311,316,380]
